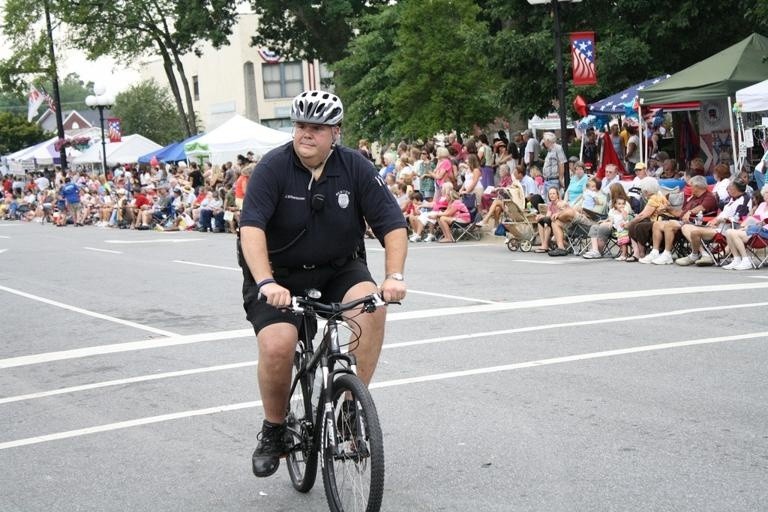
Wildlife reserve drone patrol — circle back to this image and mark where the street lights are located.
[84,94,118,180]
[527,0,584,195]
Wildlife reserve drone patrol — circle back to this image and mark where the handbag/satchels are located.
[445,176,461,189]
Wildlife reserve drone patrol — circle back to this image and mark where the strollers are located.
[503,198,557,251]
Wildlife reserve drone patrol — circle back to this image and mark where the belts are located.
[542,177,561,181]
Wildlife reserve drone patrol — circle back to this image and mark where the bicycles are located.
[281,294,402,512]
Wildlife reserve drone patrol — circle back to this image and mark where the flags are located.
[26,84,44,123]
[570,32,596,85]
[106,118,122,143]
[38,85,57,112]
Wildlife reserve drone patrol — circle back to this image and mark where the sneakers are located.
[722,256,741,270]
[548,248,568,256]
[650,253,674,265]
[732,256,753,270]
[695,255,715,266]
[638,251,659,265]
[539,216,554,224]
[615,256,628,260]
[675,254,700,266]
[583,249,602,259]
[104,221,239,234]
[410,233,456,243]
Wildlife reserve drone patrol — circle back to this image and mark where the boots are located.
[336,400,363,443]
[253,420,292,476]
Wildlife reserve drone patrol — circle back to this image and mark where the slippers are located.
[625,256,640,262]
[533,247,551,253]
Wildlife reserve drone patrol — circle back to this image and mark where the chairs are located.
[566,193,768,270]
[432,192,481,242]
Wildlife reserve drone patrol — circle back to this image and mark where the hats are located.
[520,129,534,138]
[633,162,647,171]
[157,184,194,195]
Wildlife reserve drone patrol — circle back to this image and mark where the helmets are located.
[290,89,343,127]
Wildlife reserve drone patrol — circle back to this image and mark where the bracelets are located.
[251,278,276,289]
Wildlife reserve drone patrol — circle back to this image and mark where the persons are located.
[0,121,260,234]
[359,123,768,270]
[235,91,417,476]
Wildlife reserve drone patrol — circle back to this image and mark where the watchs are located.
[387,272,408,281]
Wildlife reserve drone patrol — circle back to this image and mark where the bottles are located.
[696,210,703,226]
[309,363,323,409]
